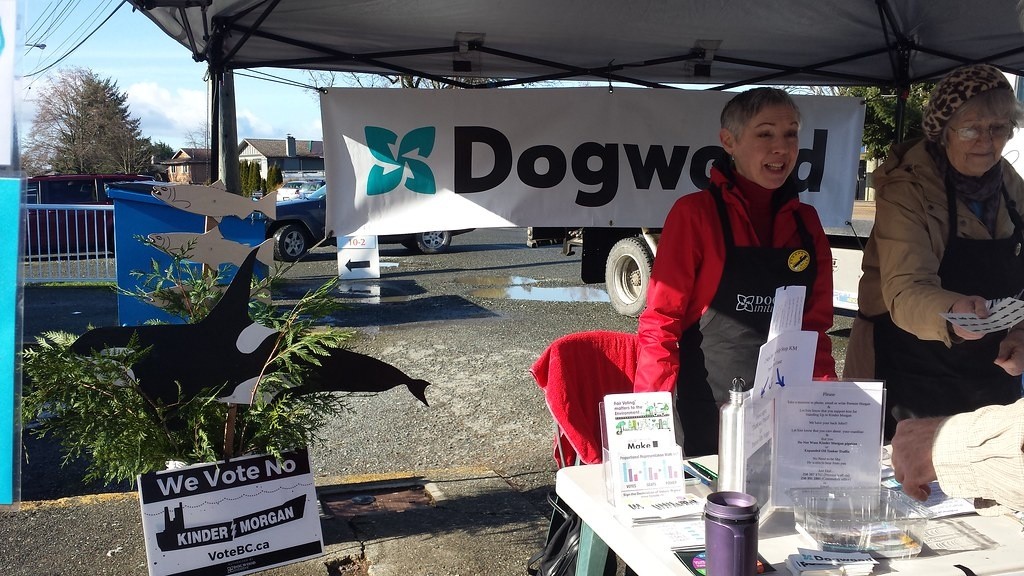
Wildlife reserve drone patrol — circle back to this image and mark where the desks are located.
[556,445,1024,576]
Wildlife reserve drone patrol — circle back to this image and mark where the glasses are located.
[944,123,1013,141]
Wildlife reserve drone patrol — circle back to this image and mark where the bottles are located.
[717,377,751,493]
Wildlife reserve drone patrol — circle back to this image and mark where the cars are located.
[276,182,308,200]
[263,181,476,262]
[22,174,157,256]
[295,181,326,199]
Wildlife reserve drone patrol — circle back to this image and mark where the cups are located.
[702,491,760,576]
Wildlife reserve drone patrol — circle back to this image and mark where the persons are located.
[633,87,838,456]
[891,398,1024,513]
[843,64,1024,441]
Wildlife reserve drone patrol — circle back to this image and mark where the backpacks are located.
[527,512,617,576]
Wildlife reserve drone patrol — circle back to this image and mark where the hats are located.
[922,64,1014,143]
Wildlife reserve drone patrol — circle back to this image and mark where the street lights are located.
[11,43,46,166]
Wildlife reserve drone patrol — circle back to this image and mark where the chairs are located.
[527,331,640,576]
